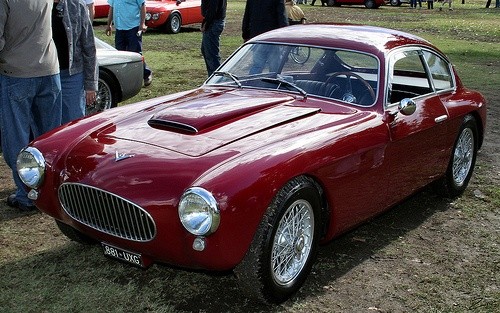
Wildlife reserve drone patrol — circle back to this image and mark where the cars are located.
[24,36,145,143]
[322,0,387,10]
[145,0,205,34]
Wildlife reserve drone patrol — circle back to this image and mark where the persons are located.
[0,0,62,211]
[201,0,227,77]
[242,0,287,73]
[296,0,500,11]
[52,0,98,124]
[106,0,152,86]
[84,0,95,23]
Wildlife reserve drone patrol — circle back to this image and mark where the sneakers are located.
[144,72,152,86]
[7,194,34,211]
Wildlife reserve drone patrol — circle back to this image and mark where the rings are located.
[92,96,94,98]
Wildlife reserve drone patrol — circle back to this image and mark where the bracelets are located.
[108,24,112,27]
[142,21,145,23]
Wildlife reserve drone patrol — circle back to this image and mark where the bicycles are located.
[264,18,310,65]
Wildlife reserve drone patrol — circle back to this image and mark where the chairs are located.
[356,88,419,106]
[289,80,340,98]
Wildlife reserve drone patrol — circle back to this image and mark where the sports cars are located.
[15,21,488,305]
